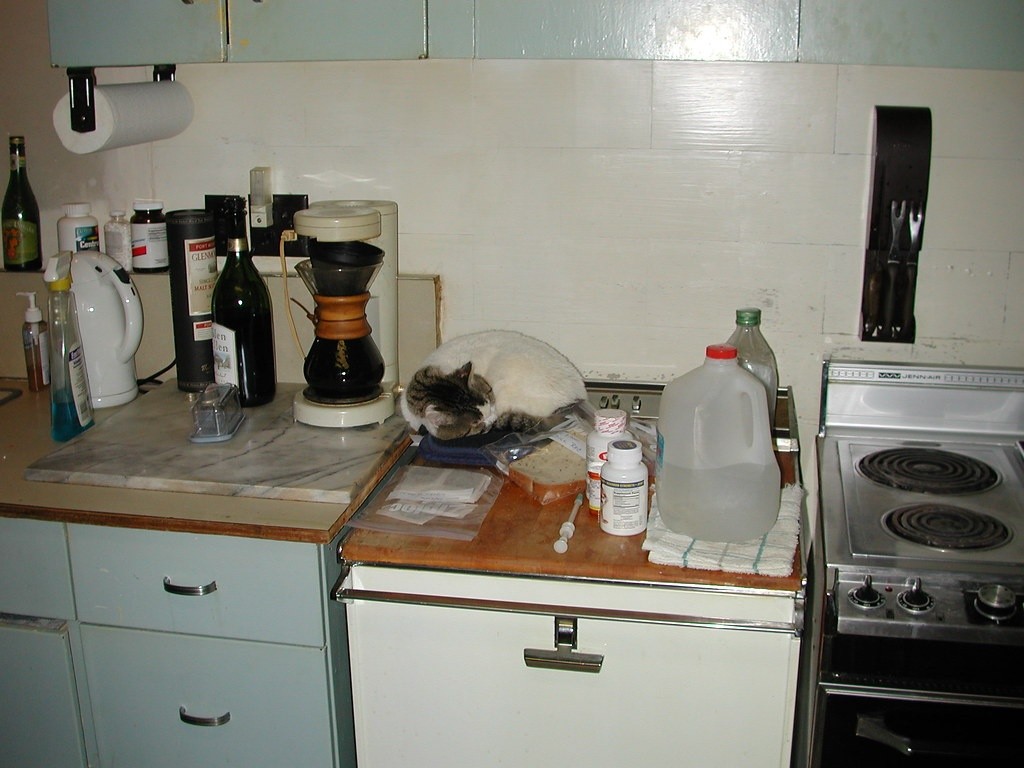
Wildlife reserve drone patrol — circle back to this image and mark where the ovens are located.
[808,595,1024,768]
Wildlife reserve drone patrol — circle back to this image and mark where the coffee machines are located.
[290,199,399,428]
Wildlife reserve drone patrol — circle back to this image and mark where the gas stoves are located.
[813,361,1023,647]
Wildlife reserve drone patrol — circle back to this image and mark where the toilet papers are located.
[52,79,196,155]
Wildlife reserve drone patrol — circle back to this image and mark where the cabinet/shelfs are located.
[0,449,426,768]
[46,0,1024,69]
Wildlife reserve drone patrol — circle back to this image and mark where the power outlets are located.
[246,193,311,257]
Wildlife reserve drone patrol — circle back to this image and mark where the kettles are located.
[50,251,144,409]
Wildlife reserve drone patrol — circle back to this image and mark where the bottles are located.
[104,210,131,271]
[655,344,781,542]
[0,136,42,272]
[211,197,276,408]
[586,409,633,515]
[726,308,779,431]
[130,198,169,274]
[600,440,648,536]
[57,203,100,254]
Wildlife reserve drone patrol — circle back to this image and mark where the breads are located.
[508,428,590,506]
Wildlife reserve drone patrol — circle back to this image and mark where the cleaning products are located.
[42,248,97,443]
[15,291,51,393]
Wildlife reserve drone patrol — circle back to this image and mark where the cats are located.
[400,328,588,448]
